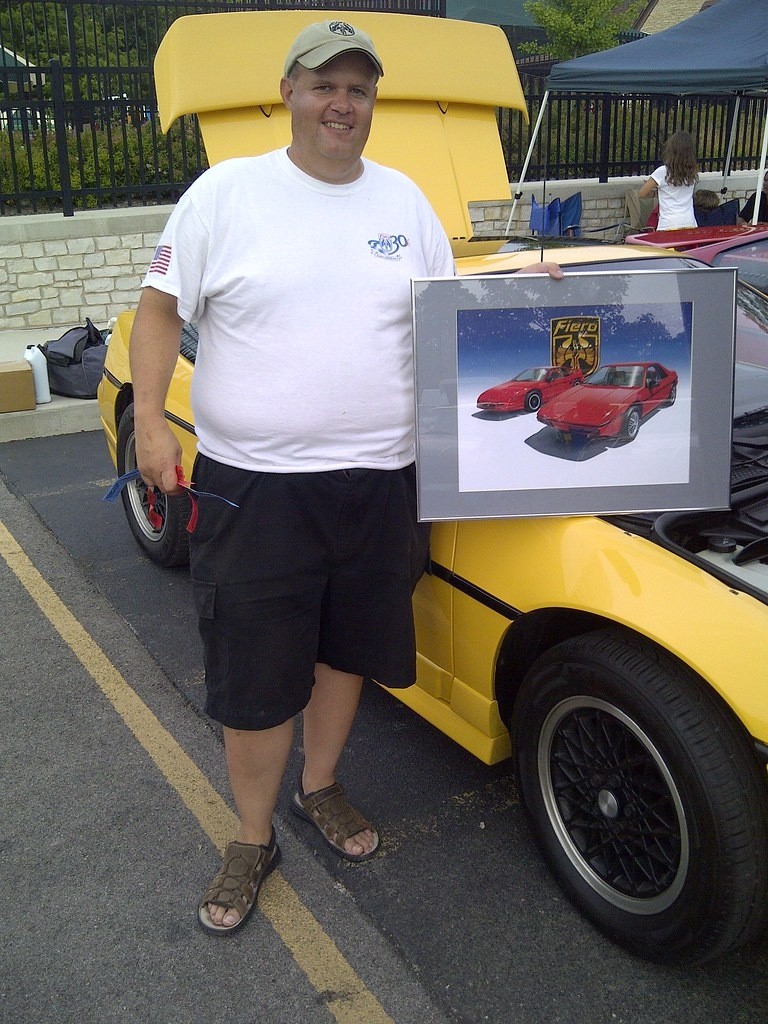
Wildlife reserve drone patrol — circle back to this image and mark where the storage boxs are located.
[0,359,36,413]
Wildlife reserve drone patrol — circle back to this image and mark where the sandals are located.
[290,774,380,862]
[197,826,280,935]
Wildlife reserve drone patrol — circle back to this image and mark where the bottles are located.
[24,344,52,403]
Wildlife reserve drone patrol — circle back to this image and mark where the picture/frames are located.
[410,268,739,521]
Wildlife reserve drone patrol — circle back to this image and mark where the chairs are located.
[696,197,741,227]
[529,191,583,237]
[611,189,660,241]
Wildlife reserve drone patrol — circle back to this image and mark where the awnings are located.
[544,1,768,183]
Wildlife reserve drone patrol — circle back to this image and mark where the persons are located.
[639,131,698,231]
[737,171,768,226]
[694,189,720,211]
[129,22,565,933]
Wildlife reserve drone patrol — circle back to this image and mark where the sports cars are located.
[98,7,768,970]
[474,366,584,420]
[536,362,679,442]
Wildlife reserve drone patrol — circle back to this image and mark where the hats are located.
[283,21,386,81]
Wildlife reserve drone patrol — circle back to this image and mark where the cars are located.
[626,223,768,374]
[0,108,70,139]
